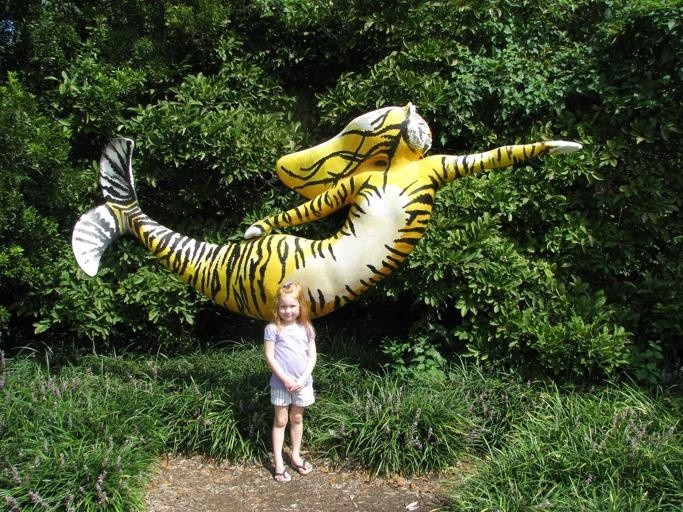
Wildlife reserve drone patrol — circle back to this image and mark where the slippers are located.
[294,460,311,475]
[275,469,290,483]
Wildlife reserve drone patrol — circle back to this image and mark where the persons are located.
[263,280,318,482]
